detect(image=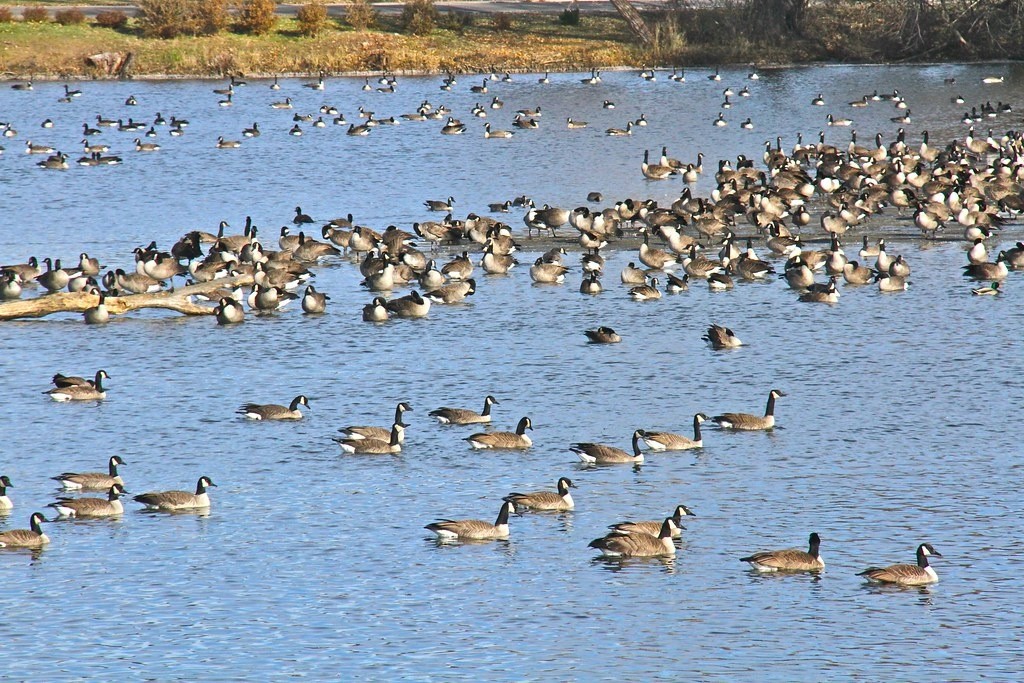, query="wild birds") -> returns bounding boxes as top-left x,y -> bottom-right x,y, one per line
0,455 -> 218,550
0,63 -> 1024,350
424,477 -> 943,586
234,387 -> 788,464
40,370 -> 112,402
